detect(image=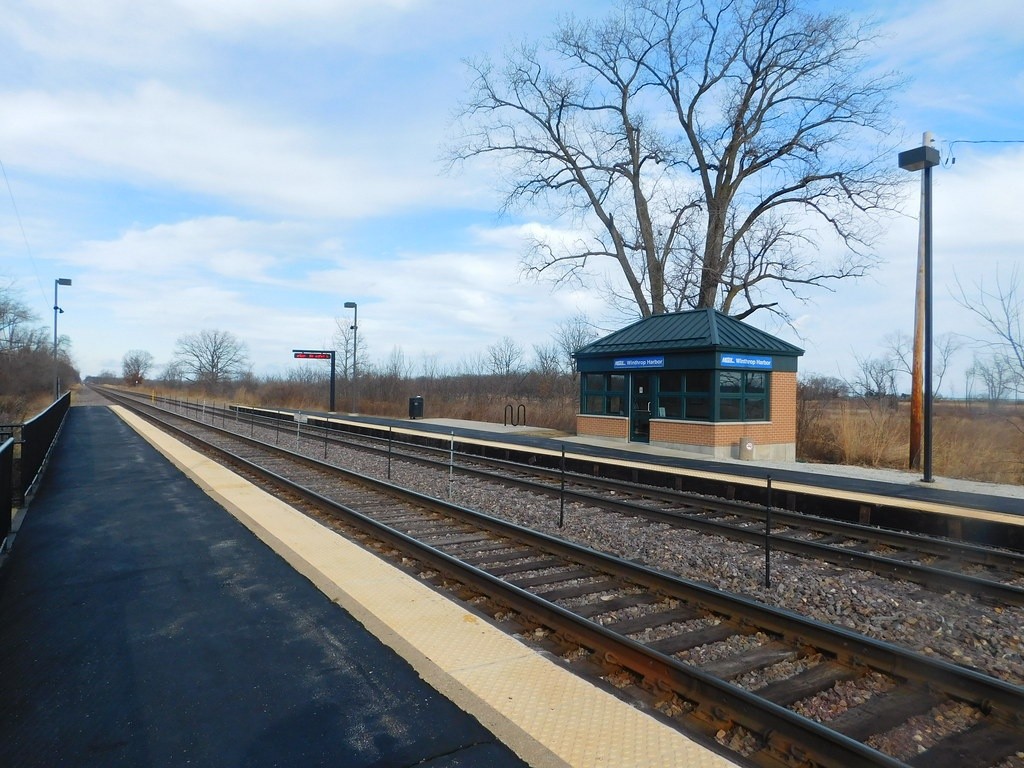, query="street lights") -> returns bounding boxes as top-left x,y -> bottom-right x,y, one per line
53,278 -> 72,402
345,301 -> 358,415
897,145 -> 940,484
